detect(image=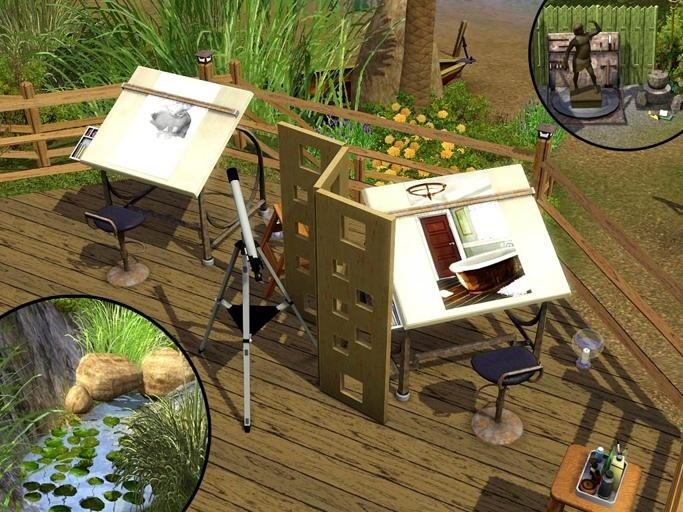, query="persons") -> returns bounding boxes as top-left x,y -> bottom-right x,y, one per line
563,20 -> 602,94
